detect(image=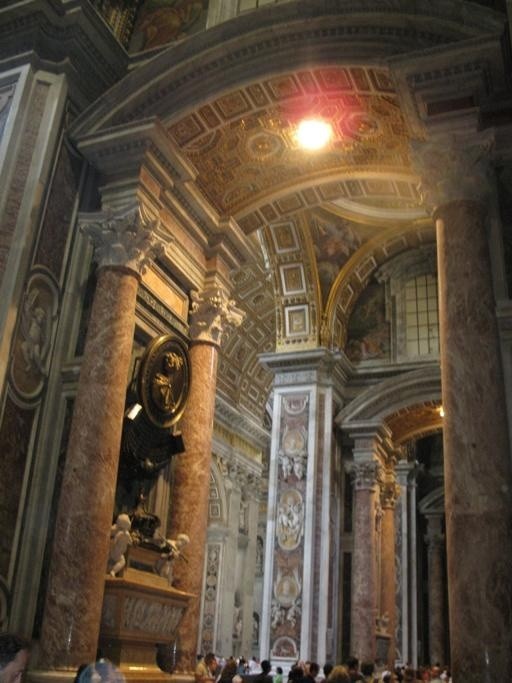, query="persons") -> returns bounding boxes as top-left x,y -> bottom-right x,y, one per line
276,500 -> 293,529
106,513 -> 138,576
268,597 -> 286,629
277,447 -> 294,478
20,286 -> 49,376
193,652 -> 453,683
286,597 -> 302,627
292,448 -> 307,479
290,499 -> 303,527
158,532 -> 190,585
2,631 -> 33,682
